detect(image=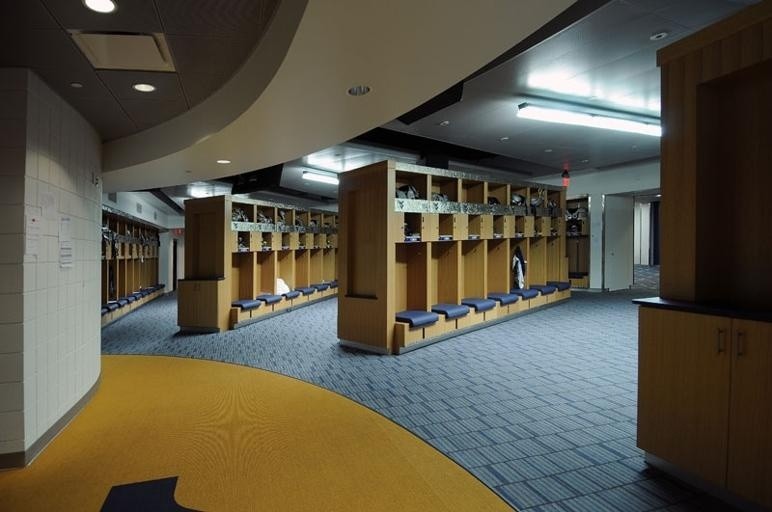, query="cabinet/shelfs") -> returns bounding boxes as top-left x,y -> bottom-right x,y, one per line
101,204 -> 165,329
634,306 -> 772,512
177,194 -> 338,333
338,159 -> 573,355
569,196 -> 591,291
